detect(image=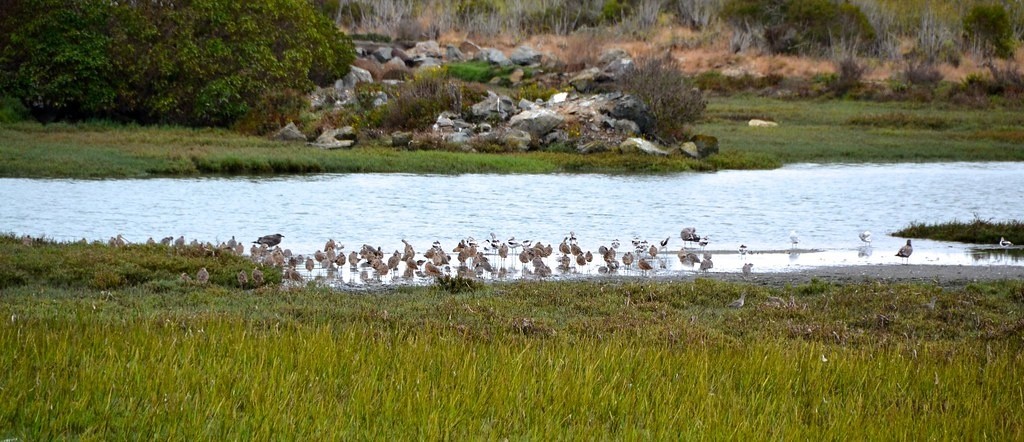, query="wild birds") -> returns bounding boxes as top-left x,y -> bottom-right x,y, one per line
922,296 -> 938,310
728,292 -> 747,309
108,228 -> 1014,287
